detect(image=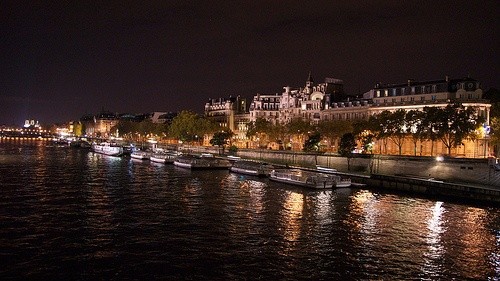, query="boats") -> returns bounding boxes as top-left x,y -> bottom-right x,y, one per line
130,150 -> 231,169
270,169 -> 352,188
229,159 -> 273,176
94,142 -> 124,157
